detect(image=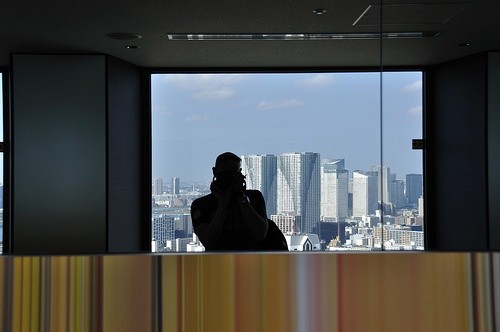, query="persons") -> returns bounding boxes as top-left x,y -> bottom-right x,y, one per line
190,151 -> 270,252
